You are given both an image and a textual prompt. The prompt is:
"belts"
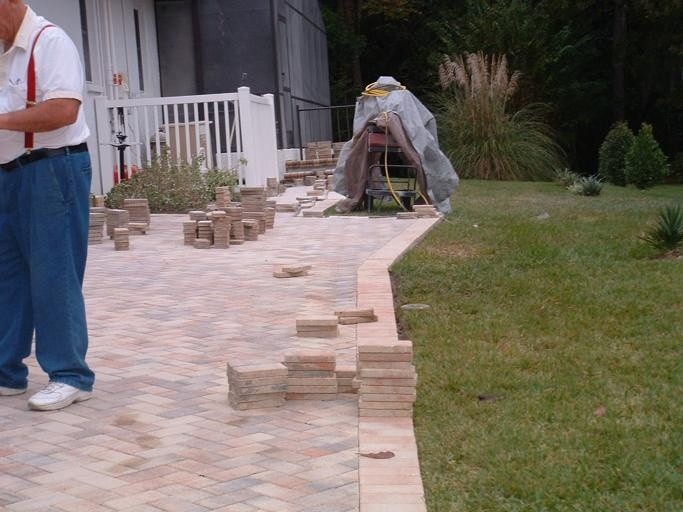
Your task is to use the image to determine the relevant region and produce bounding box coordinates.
[0,143,88,171]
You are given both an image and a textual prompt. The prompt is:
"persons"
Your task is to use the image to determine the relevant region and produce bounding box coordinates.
[0,0,96,411]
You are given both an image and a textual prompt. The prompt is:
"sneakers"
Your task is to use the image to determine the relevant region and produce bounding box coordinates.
[0,386,26,396]
[27,382,94,411]
